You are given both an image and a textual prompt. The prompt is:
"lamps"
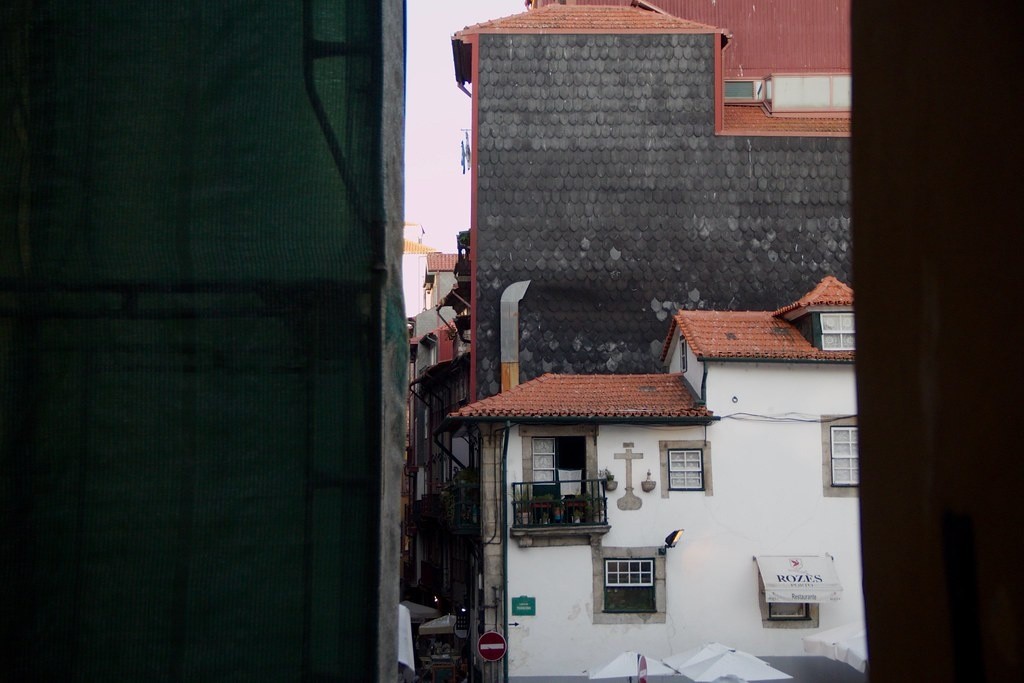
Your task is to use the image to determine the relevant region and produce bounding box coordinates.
[658,529,684,555]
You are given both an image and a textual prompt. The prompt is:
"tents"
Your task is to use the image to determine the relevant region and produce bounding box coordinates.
[418,615,457,635]
[399,600,440,623]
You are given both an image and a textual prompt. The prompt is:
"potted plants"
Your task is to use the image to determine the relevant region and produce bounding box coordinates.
[508,468,618,526]
[443,470,478,525]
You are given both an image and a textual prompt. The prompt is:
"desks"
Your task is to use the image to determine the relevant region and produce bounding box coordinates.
[431,661,455,683]
